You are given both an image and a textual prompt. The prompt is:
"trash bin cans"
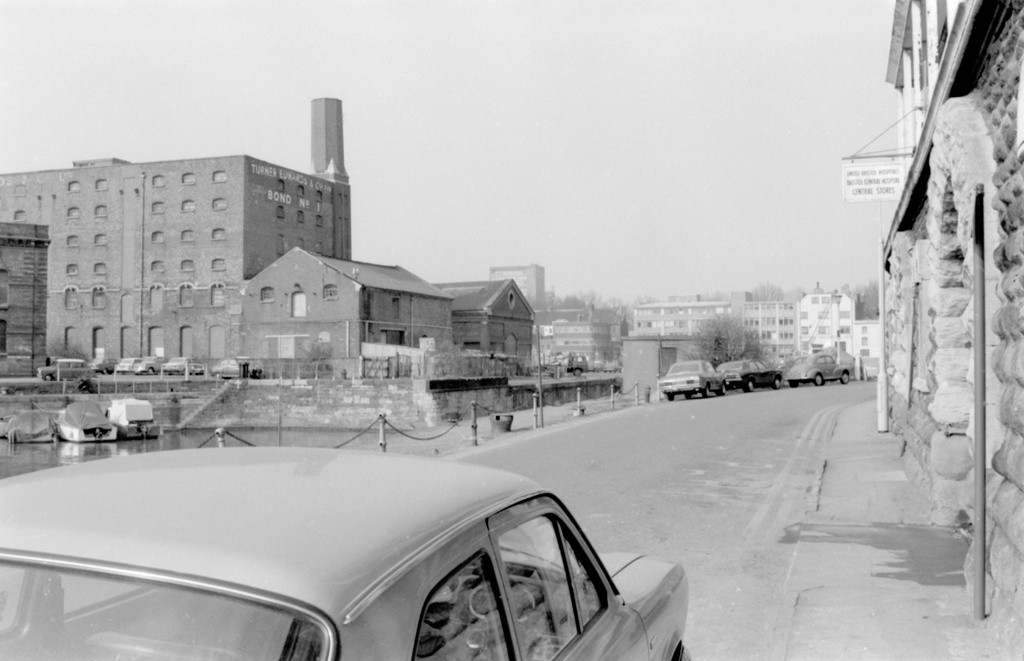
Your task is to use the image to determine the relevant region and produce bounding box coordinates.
[489,414,514,436]
[340,369,347,379]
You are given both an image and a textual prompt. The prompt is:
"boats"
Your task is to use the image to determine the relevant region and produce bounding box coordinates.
[4,409,57,449]
[53,400,119,442]
[104,398,160,440]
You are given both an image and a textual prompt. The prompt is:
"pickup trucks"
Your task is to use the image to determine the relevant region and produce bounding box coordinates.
[556,354,588,377]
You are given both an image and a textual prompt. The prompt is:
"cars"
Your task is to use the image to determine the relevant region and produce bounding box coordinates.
[36,358,94,381]
[1,445,689,660]
[91,357,118,374]
[602,362,617,374]
[784,354,852,388]
[210,357,259,381]
[658,360,726,401]
[716,359,784,393]
[114,357,145,376]
[162,357,206,374]
[141,356,171,374]
